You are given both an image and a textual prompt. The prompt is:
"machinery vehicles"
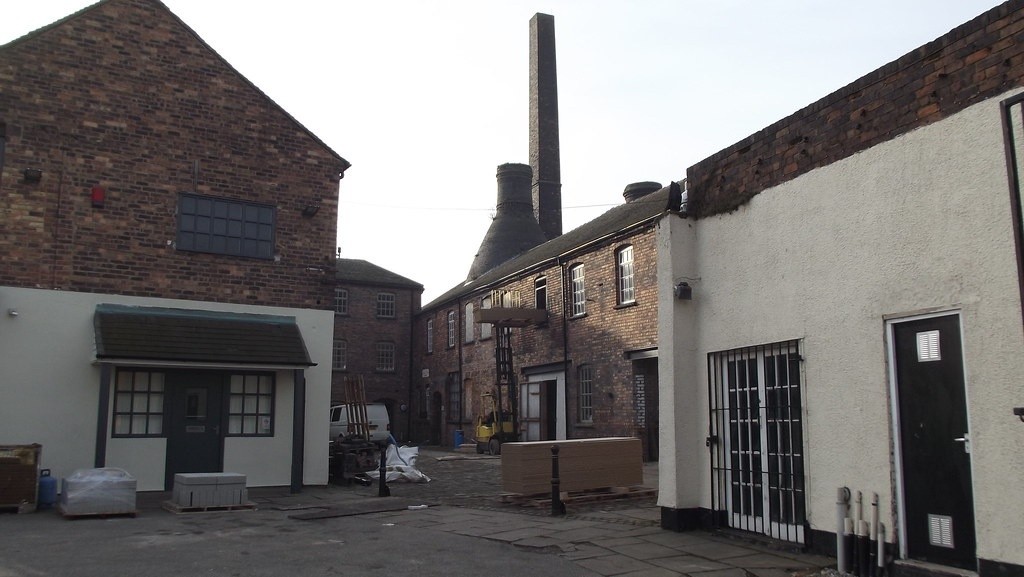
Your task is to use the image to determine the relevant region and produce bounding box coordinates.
[473,322,521,455]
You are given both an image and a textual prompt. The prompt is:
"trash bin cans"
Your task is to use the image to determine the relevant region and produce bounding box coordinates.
[454,429,464,449]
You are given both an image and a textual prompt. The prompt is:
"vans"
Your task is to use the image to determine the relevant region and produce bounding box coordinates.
[329,404,390,448]
